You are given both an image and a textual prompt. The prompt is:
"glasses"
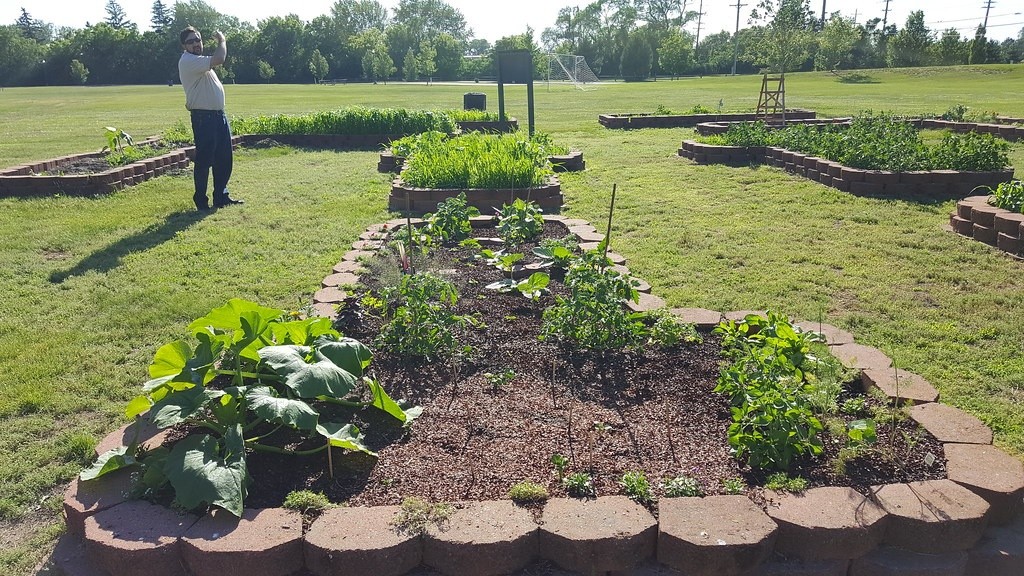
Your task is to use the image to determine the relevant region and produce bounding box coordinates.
[184,38,202,44]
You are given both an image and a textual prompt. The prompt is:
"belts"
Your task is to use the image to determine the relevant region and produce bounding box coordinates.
[191,110,224,115]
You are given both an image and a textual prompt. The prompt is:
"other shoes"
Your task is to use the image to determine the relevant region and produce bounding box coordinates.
[228,199,243,205]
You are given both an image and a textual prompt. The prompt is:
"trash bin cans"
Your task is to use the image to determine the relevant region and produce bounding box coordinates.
[475,78,479,83]
[464,92,486,111]
[168,81,173,86]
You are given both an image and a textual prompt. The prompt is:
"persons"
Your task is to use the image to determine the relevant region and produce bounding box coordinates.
[177,25,243,209]
